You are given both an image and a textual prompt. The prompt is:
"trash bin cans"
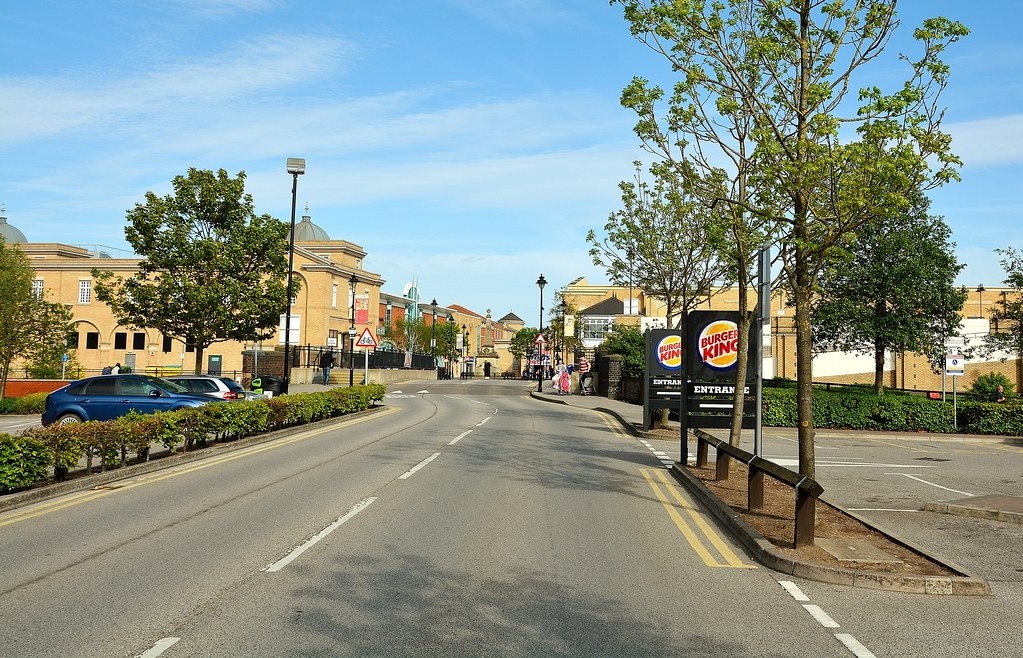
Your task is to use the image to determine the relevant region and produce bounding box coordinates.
[251,375,283,397]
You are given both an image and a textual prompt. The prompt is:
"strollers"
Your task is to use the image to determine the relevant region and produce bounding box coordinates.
[578,371,598,396]
[558,372,572,395]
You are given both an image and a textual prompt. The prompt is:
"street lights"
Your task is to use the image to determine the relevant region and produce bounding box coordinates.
[281,156,306,397]
[449,314,455,380]
[465,332,470,380]
[536,273,548,393]
[462,324,466,380]
[429,297,438,370]
[347,272,361,388]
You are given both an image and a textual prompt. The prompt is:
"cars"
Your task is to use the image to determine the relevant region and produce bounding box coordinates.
[249,375,283,396]
[122,375,237,403]
[40,373,229,430]
[186,374,268,401]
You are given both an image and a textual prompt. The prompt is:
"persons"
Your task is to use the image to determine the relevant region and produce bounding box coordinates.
[990,385,1007,404]
[510,371,515,380]
[552,358,571,389]
[503,370,510,380]
[111,362,121,374]
[521,370,528,380]
[319,350,334,385]
[536,369,540,380]
[571,353,591,392]
